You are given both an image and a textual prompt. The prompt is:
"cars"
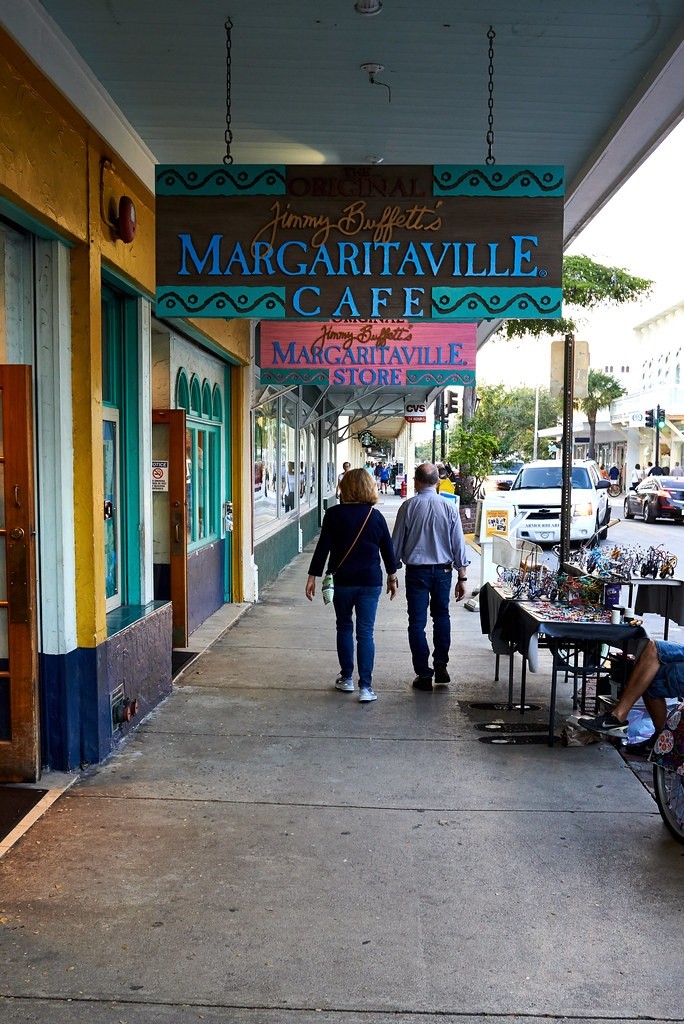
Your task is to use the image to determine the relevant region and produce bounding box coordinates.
[454,470,474,486]
[273,464,286,481]
[624,476,684,526]
[488,461,525,487]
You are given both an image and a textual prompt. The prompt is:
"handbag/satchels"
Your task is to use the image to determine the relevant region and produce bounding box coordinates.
[638,478,642,483]
[322,574,334,605]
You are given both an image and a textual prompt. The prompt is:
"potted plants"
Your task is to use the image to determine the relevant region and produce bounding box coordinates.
[446,429,499,533]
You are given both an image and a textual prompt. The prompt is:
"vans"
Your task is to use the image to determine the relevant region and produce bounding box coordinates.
[496,458,612,550]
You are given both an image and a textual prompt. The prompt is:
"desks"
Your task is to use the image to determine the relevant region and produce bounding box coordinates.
[468,561,681,747]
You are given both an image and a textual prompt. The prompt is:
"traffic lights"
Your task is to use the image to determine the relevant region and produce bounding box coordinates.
[645,409,653,428]
[658,409,665,428]
[444,414,449,423]
[447,390,458,414]
[434,416,440,430]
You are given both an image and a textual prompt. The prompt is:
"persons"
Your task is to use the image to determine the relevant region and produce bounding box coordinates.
[577,636,684,756]
[616,464,625,494]
[335,462,351,505]
[669,460,684,477]
[599,465,610,480]
[305,467,399,700]
[631,464,643,493]
[661,451,670,476]
[607,463,619,495]
[282,461,293,513]
[298,462,306,497]
[362,461,395,494]
[437,462,456,498]
[644,462,654,476]
[644,444,651,466]
[390,463,469,688]
[648,461,663,477]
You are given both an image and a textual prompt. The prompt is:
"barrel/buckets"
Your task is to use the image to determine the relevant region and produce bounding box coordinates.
[608,651,636,702]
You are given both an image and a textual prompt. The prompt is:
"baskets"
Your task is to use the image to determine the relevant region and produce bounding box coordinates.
[492,533,543,569]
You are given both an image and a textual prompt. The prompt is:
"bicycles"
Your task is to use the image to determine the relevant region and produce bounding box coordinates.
[604,477,621,497]
[551,518,621,675]
[646,702,684,842]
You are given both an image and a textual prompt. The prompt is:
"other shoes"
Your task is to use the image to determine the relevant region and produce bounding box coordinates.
[435,667,451,683]
[412,673,433,691]
[381,491,383,494]
[385,492,387,494]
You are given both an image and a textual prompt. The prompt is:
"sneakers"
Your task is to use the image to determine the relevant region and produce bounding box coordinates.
[358,686,378,701]
[578,712,630,737]
[625,732,662,756]
[335,676,355,691]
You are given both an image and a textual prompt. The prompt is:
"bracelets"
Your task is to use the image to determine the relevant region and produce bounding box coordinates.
[457,575,468,581]
[387,577,397,582]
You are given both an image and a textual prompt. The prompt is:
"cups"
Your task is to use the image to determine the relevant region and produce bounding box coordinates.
[611,610,620,624]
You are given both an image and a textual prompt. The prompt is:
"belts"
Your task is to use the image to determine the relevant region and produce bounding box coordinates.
[410,564,452,570]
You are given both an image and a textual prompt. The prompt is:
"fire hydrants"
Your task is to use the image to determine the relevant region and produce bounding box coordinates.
[400,481,407,498]
